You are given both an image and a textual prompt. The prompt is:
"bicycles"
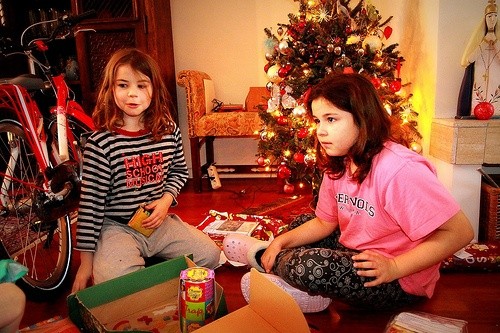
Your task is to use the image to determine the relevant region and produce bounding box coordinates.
[0,9,99,302]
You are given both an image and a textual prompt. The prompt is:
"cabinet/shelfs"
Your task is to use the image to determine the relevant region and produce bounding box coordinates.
[429,117,500,165]
[26,0,179,132]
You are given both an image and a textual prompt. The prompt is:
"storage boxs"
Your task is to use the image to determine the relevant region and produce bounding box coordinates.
[481,174,500,241]
[66,254,311,333]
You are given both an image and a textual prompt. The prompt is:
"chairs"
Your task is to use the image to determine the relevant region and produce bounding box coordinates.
[177,70,273,193]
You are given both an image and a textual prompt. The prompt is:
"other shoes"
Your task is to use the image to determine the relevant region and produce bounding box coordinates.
[223,233,270,273]
[240,272,330,314]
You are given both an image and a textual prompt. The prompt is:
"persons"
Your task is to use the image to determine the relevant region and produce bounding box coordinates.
[223,72,474,312]
[71,47,221,295]
[455,0,500,120]
[0,283,26,333]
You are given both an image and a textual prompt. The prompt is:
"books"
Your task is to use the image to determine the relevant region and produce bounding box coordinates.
[203,220,260,237]
